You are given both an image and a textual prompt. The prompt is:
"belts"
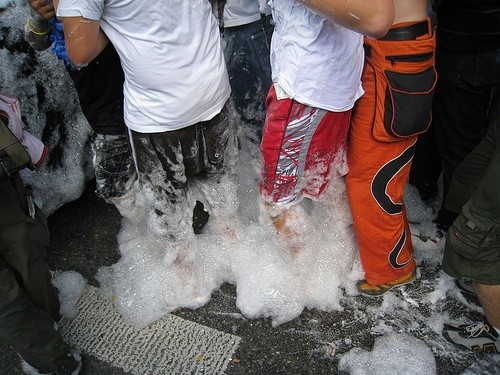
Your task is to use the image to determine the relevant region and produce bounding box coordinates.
[377,18,436,40]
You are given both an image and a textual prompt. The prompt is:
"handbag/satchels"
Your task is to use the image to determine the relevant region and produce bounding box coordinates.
[370,48,437,142]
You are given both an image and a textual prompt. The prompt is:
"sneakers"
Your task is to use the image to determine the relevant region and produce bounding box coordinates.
[441,315,500,353]
[455,278,482,308]
[356,266,417,296]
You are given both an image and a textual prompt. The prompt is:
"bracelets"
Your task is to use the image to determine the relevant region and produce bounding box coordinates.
[27,18,51,36]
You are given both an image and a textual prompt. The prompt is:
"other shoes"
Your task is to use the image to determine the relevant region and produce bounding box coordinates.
[52,271,84,324]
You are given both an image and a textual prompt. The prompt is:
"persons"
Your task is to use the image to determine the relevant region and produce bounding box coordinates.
[207,1,276,146]
[258,1,394,254]
[0,120,82,375]
[25,0,138,203]
[56,1,241,242]
[410,0,500,233]
[442,101,500,354]
[344,1,437,296]
